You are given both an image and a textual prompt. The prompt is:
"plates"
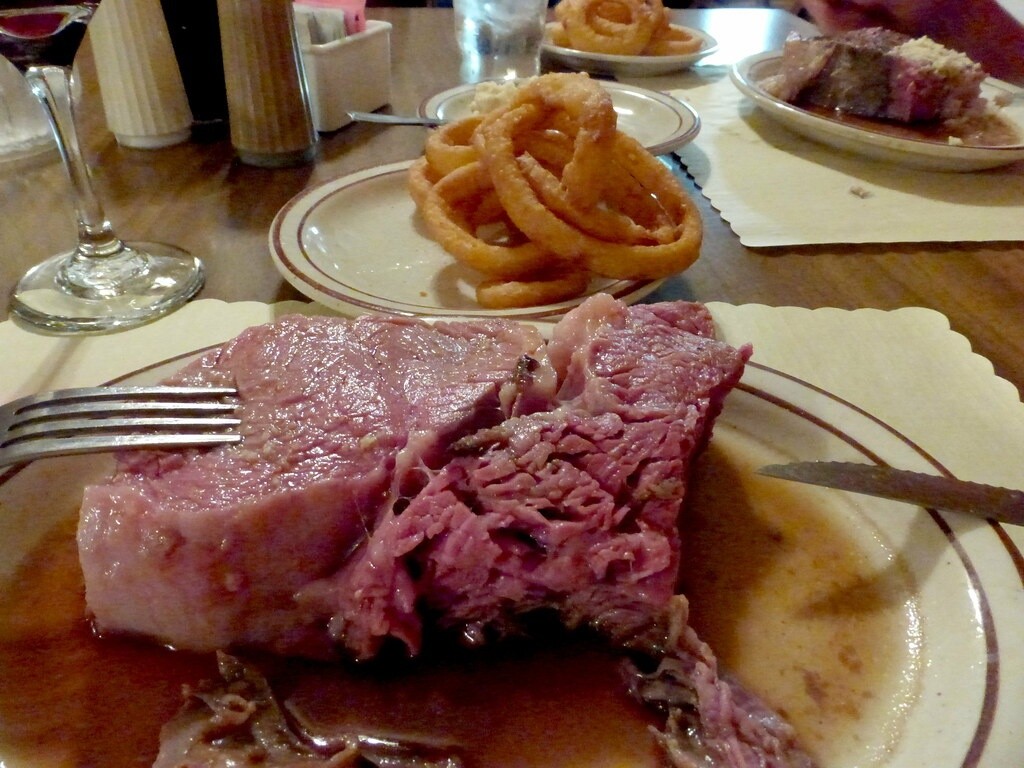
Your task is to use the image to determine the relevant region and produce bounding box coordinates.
[728,48,1024,174]
[418,81,701,161]
[0,328,1024,768]
[541,27,721,74]
[269,158,679,330]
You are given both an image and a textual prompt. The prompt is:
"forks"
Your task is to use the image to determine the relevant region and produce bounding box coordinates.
[1,379,245,485]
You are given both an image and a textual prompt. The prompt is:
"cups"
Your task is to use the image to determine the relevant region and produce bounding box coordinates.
[451,0,548,85]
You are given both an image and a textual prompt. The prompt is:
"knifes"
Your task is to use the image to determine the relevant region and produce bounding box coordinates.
[752,459,1024,531]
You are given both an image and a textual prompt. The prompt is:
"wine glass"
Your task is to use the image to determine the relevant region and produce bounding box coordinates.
[0,0,205,331]
